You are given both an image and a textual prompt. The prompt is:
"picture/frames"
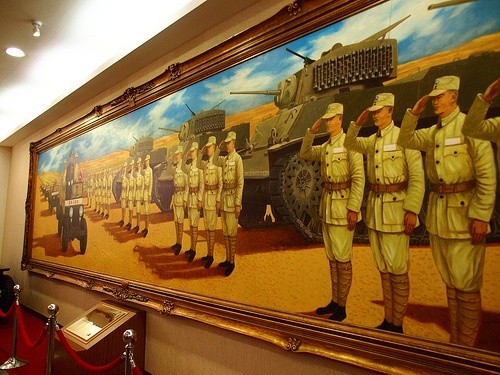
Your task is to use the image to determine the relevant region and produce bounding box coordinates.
[21,0,500,375]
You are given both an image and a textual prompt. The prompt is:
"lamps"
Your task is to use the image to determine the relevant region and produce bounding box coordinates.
[32,21,42,37]
